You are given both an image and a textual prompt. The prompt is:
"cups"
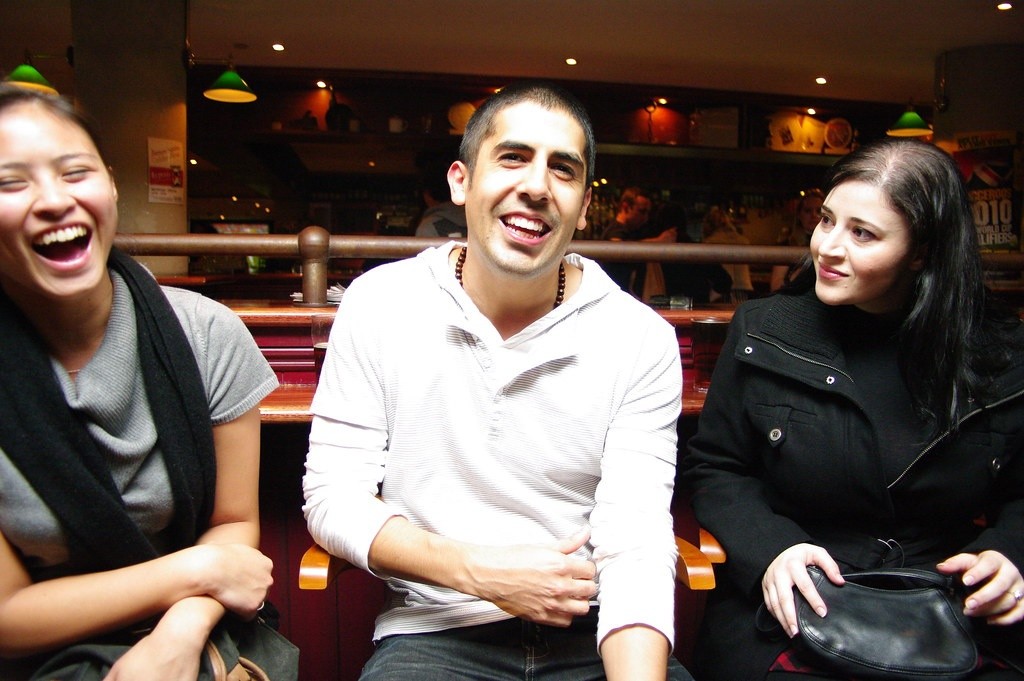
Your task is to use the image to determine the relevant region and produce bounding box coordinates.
[689,317,732,394]
[311,315,335,385]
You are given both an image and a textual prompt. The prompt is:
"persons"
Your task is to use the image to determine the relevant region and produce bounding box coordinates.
[700,211,754,303]
[770,189,825,292]
[304,86,696,681]
[686,139,1024,681]
[415,165,467,238]
[0,87,278,681]
[189,221,248,273]
[596,188,677,293]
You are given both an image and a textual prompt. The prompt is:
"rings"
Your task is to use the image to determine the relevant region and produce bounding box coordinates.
[257,602,264,610]
[1006,591,1023,607]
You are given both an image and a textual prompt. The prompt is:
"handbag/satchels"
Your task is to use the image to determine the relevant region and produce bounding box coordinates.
[31,615,300,681]
[756,559,979,681]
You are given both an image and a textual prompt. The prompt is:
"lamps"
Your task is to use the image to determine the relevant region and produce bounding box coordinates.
[190,55,258,103]
[886,96,936,137]
[2,44,74,98]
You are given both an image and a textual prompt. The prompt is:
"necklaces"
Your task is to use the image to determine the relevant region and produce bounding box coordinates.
[68,370,80,373]
[455,246,566,307]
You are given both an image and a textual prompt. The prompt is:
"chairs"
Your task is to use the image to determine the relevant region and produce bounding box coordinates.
[296,518,731,680]
